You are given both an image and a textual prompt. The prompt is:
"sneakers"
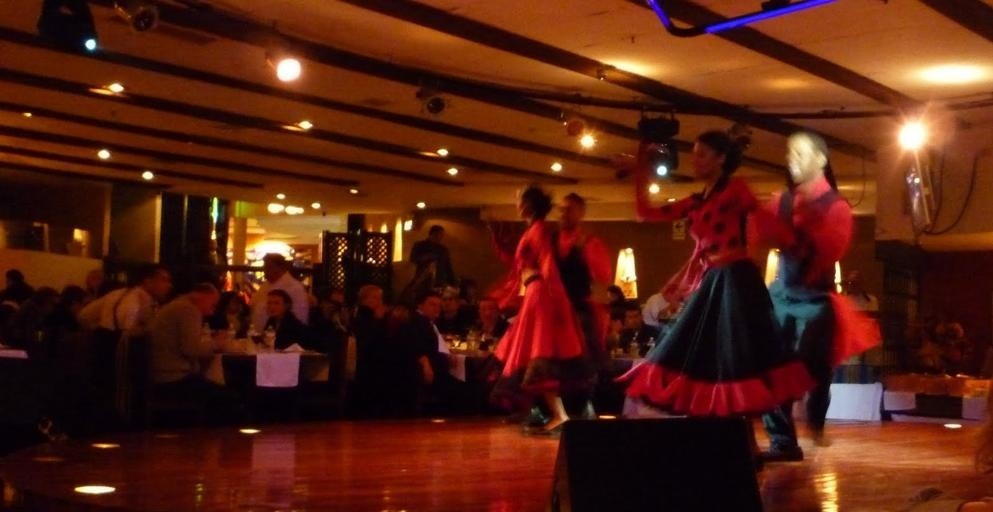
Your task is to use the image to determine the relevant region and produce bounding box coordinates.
[758,443,803,461]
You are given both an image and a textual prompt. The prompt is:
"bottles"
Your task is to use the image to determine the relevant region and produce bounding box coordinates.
[442,327,493,351]
[627,331,656,357]
[217,322,276,350]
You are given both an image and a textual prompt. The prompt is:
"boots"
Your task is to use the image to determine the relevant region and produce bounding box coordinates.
[542,396,571,430]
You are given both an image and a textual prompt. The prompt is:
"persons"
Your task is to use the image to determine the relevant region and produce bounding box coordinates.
[1,224,513,464]
[493,128,881,462]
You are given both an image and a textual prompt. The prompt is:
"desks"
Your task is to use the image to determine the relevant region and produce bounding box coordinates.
[214,338,326,424]
[445,348,492,383]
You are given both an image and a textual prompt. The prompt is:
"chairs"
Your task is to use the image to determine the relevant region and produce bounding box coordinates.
[115,328,205,432]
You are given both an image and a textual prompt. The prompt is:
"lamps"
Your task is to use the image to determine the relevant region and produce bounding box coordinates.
[113,0,160,34]
[262,35,301,83]
[636,111,679,177]
[416,87,447,116]
[560,109,584,136]
